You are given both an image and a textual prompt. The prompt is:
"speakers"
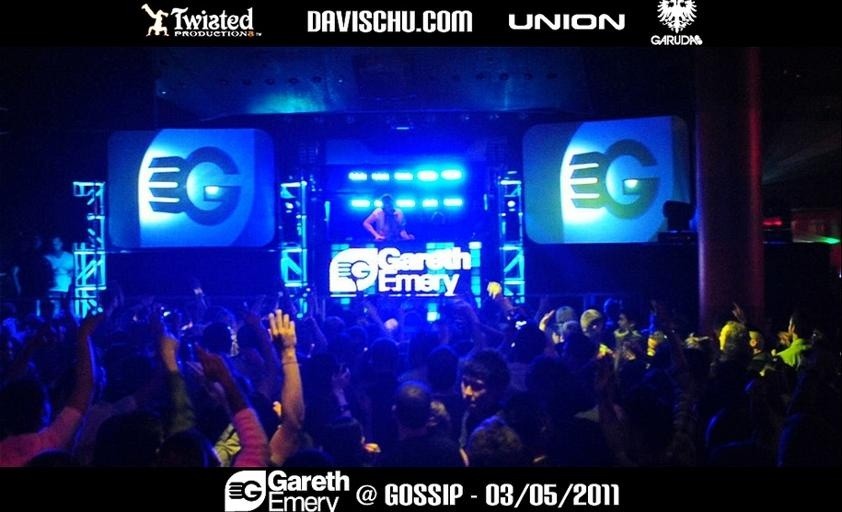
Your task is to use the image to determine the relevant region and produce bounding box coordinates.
[657,229,699,336]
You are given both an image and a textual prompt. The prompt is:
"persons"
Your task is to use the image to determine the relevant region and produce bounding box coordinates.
[45,236,77,301]
[363,194,416,241]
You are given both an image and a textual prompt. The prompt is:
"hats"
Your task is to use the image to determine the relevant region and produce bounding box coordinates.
[580,309,602,328]
